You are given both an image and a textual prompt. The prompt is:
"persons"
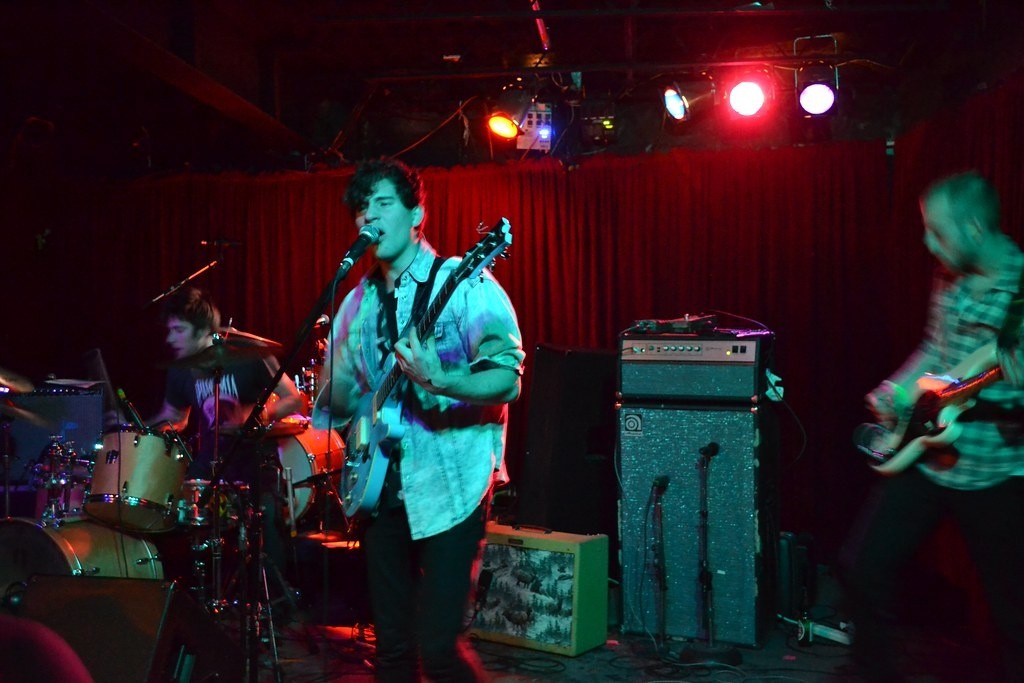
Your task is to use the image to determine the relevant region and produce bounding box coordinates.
[833,170,1024,683]
[158,286,303,644]
[312,158,526,683]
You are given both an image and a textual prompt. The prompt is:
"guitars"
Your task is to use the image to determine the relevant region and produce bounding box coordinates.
[340,216,515,523]
[865,364,1005,475]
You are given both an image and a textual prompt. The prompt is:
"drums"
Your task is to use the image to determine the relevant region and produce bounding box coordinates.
[0,514,166,603]
[84,424,192,536]
[256,415,348,535]
[181,479,245,527]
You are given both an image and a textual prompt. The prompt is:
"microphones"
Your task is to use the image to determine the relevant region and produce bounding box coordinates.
[201,241,242,250]
[336,224,379,280]
[284,468,297,538]
[653,476,669,488]
[117,388,148,433]
[699,442,720,456]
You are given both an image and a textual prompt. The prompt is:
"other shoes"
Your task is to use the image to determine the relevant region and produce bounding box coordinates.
[774,592,863,647]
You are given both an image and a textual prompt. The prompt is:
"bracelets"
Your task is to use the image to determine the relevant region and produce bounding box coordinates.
[883,380,906,398]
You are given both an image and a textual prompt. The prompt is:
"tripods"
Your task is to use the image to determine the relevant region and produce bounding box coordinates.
[216,445,320,683]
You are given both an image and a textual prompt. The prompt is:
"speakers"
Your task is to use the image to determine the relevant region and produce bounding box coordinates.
[466,518,609,656]
[0,383,105,486]
[614,401,781,650]
[517,343,621,587]
[17,573,244,683]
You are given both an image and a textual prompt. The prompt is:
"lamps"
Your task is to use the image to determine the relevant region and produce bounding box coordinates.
[485,77,540,139]
[796,68,836,116]
[730,70,776,116]
[663,72,721,121]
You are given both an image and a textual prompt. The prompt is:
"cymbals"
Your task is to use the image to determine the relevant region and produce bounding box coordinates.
[216,325,284,351]
